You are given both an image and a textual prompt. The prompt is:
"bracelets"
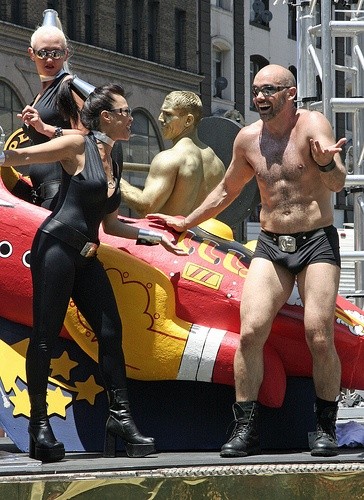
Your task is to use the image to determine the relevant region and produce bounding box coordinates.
[52,126,64,140]
[0,152,6,166]
[320,159,337,173]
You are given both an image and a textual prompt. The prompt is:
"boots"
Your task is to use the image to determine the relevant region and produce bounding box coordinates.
[27,392,66,460]
[310,398,339,457]
[101,386,158,458]
[219,402,263,457]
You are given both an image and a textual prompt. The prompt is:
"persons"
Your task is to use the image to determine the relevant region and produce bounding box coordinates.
[115,90,224,219]
[145,65,347,456]
[0,24,192,462]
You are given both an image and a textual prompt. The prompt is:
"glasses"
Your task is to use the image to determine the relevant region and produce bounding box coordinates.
[110,106,132,118]
[253,85,294,97]
[29,44,70,60]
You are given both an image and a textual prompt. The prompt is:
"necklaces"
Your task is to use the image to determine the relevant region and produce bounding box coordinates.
[103,144,114,190]
